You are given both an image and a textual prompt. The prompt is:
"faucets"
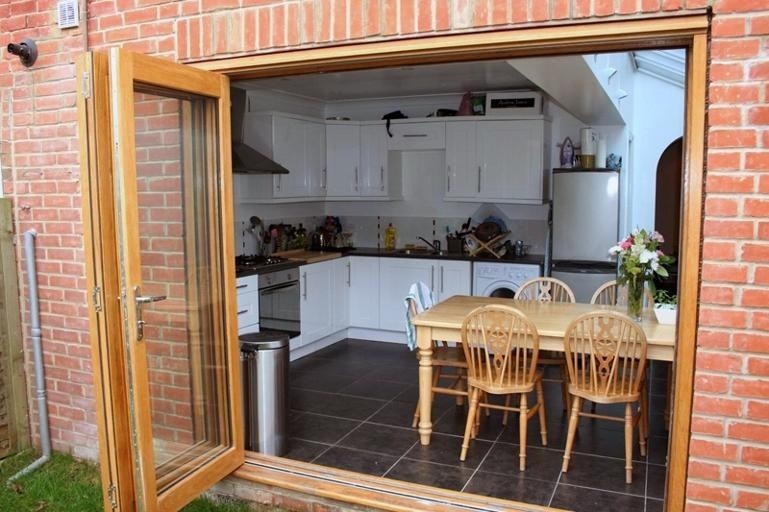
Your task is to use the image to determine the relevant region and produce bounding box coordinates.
[417,236,440,253]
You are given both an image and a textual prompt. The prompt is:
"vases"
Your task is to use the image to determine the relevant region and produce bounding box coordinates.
[625,278,643,323]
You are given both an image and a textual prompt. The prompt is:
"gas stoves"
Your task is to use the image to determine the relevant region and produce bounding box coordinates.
[235,254,299,288]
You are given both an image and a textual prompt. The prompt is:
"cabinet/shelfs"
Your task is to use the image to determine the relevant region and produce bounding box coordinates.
[378,253,472,334]
[327,118,360,201]
[346,255,379,331]
[236,274,260,336]
[443,115,478,203]
[243,112,325,205]
[480,112,551,206]
[388,118,443,153]
[298,256,347,352]
[363,118,401,201]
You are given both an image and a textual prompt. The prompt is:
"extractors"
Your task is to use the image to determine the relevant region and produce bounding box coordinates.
[228,85,289,176]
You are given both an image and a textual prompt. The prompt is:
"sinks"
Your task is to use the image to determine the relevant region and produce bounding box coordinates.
[392,249,444,256]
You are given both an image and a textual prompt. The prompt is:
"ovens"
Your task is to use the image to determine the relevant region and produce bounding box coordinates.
[258,280,300,342]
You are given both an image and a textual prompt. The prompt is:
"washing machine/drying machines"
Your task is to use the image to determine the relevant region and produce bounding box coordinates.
[472,261,542,349]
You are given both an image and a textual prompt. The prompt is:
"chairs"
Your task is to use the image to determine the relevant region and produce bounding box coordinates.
[559,309,650,486]
[565,279,655,441]
[400,281,492,431]
[499,278,577,428]
[455,302,549,472]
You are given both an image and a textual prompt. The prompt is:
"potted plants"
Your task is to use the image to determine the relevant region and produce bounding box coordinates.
[651,288,678,328]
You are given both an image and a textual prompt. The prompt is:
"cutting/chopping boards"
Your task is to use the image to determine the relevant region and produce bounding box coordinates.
[271,249,342,263]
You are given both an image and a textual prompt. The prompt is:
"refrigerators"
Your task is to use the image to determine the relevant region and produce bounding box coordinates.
[549,167,620,306]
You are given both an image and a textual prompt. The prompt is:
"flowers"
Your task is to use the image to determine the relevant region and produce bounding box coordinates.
[607,224,677,316]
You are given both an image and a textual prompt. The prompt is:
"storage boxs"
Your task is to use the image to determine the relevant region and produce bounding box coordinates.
[485,91,549,116]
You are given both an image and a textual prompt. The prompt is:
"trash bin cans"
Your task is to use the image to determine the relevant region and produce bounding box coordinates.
[239,332,289,457]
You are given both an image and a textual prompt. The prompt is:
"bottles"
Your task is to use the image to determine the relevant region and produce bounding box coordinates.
[458,91,472,116]
[280,231,288,250]
[515,240,523,256]
